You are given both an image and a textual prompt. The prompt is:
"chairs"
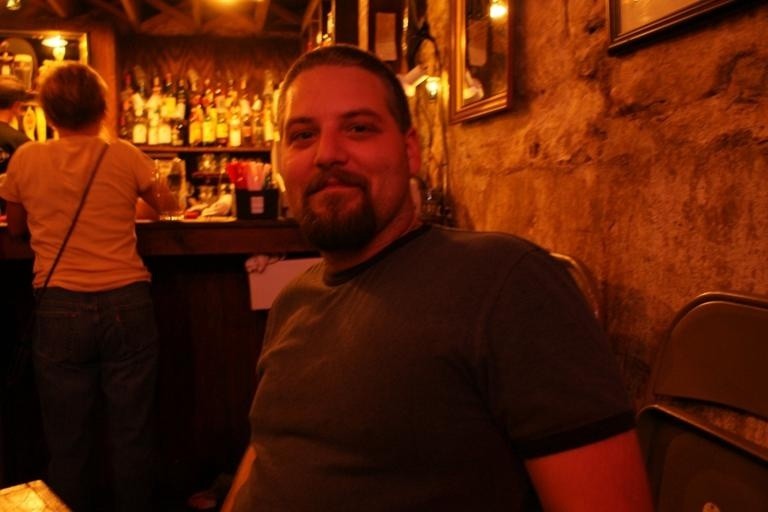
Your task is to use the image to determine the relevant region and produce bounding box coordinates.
[637,290,768,511]
[554,251,604,329]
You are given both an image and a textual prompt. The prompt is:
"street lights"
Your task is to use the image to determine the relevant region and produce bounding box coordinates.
[2,301,37,388]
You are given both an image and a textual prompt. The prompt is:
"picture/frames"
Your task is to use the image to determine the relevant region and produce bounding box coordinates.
[0,27,90,107]
[447,0,513,126]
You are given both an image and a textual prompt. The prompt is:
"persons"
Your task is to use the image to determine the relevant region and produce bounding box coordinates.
[2,66,180,512]
[221,42,654,512]
[2,74,32,173]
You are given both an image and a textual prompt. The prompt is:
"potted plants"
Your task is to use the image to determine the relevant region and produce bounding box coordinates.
[0,27,90,107]
[447,0,513,126]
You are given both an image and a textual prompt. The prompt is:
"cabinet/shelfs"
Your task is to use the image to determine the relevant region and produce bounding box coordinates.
[122,69,271,215]
[13,55,34,91]
